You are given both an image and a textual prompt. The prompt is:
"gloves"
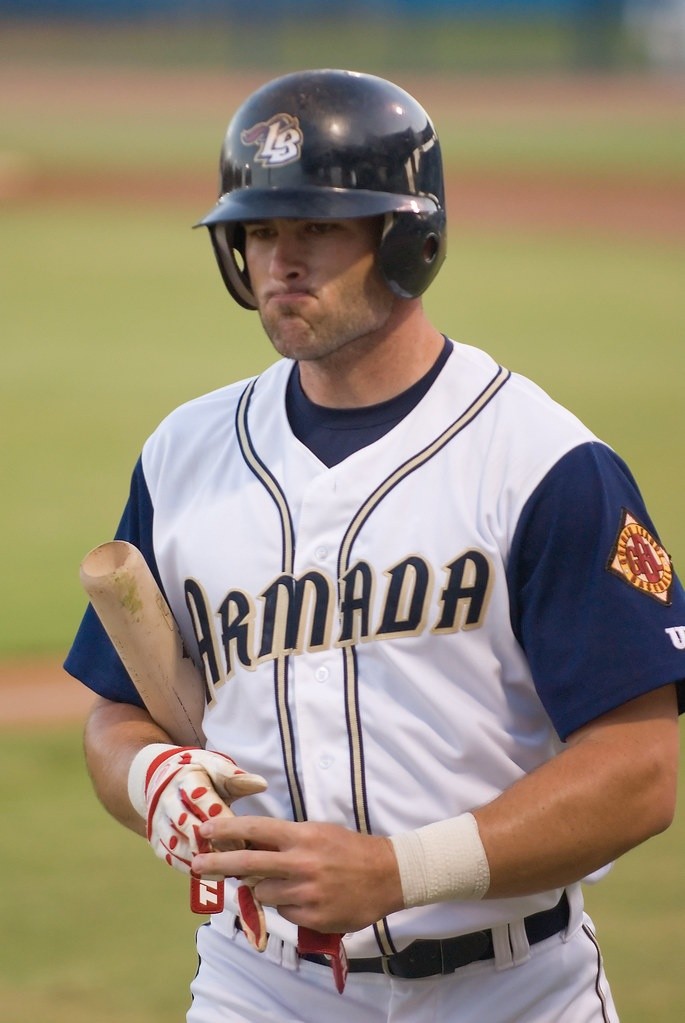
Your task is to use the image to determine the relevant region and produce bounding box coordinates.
[128,743,267,882]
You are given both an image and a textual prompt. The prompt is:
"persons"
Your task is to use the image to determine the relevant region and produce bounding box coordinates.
[64,69,685,1023]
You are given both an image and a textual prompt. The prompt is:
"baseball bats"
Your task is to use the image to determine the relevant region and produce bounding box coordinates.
[76,538,211,751]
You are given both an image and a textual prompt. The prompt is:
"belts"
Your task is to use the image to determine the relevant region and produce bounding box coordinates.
[235,889,571,977]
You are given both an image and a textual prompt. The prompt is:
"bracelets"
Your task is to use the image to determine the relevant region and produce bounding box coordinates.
[386,812,491,909]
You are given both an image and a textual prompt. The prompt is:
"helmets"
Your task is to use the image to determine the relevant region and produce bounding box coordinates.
[191,66,447,312]
